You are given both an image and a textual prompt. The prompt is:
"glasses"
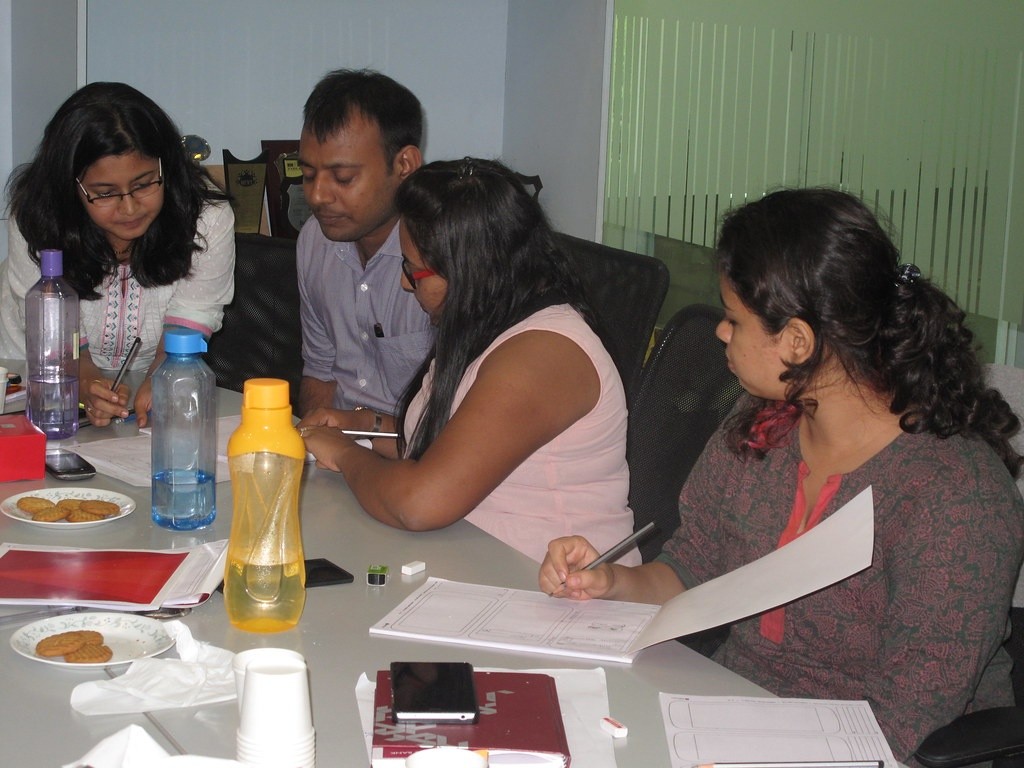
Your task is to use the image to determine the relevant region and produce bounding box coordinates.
[74,155,163,208]
[400,258,435,289]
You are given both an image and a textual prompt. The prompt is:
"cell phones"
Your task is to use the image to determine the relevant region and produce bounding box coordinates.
[217,558,354,594]
[390,662,479,726]
[43,447,96,480]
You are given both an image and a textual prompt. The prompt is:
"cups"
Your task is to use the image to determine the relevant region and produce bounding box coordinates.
[231,647,317,768]
[0,367,8,414]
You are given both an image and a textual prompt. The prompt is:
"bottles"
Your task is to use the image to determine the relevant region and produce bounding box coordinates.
[223,377,307,634]
[24,248,80,440]
[151,328,217,532]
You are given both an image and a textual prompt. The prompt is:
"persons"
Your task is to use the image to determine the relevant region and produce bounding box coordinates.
[0,82,234,424]
[540,189,1024,768]
[295,71,431,413]
[295,156,642,567]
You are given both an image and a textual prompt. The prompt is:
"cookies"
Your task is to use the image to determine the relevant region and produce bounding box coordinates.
[17,496,119,523]
[36,630,114,663]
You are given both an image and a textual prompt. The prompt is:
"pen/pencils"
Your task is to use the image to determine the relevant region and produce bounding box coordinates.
[111,337,141,392]
[342,430,398,437]
[374,323,384,338]
[115,411,151,424]
[692,760,884,768]
[549,522,655,595]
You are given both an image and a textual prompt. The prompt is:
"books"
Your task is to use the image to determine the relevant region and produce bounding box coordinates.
[371,669,571,768]
[368,484,874,664]
[0,540,227,611]
[138,414,373,472]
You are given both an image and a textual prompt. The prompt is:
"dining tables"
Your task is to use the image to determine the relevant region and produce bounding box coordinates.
[0,360,912,768]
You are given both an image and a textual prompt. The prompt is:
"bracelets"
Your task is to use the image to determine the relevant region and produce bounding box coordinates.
[368,411,382,439]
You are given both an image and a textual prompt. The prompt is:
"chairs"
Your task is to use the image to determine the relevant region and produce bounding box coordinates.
[552,230,669,384]
[913,607,1024,768]
[620,303,747,658]
[200,232,304,417]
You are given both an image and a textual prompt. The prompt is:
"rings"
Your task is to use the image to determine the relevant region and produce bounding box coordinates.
[300,428,304,436]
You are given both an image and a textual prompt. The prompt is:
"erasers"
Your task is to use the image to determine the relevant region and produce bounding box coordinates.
[600,717,628,738]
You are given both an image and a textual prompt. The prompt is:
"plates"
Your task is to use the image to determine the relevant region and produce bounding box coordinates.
[10,612,176,672]
[0,487,137,530]
